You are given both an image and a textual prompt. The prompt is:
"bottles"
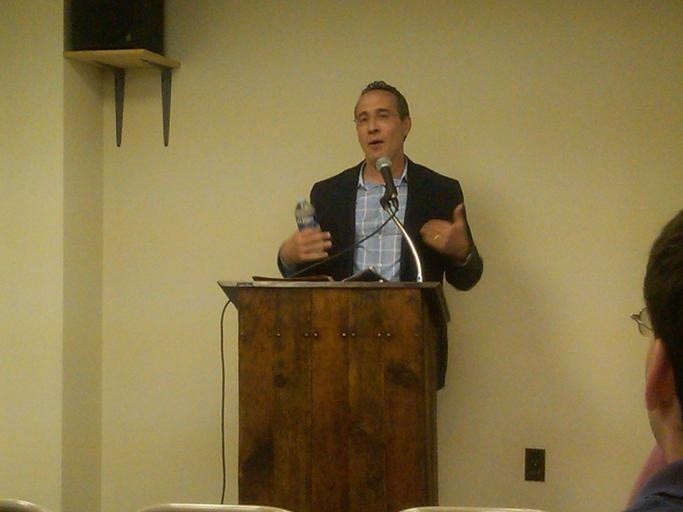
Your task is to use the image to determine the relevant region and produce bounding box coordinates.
[293,196,320,231]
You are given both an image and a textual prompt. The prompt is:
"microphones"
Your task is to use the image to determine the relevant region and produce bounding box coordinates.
[375,156,399,209]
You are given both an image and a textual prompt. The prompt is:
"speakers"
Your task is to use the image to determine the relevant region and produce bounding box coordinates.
[71,0,165,54]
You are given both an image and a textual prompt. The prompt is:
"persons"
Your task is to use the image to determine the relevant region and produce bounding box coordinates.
[618,208,683,510]
[272,80,483,294]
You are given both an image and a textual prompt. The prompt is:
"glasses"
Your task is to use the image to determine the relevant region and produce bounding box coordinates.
[628,305,655,339]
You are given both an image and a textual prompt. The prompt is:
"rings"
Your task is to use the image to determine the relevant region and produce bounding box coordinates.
[433,234,441,240]
